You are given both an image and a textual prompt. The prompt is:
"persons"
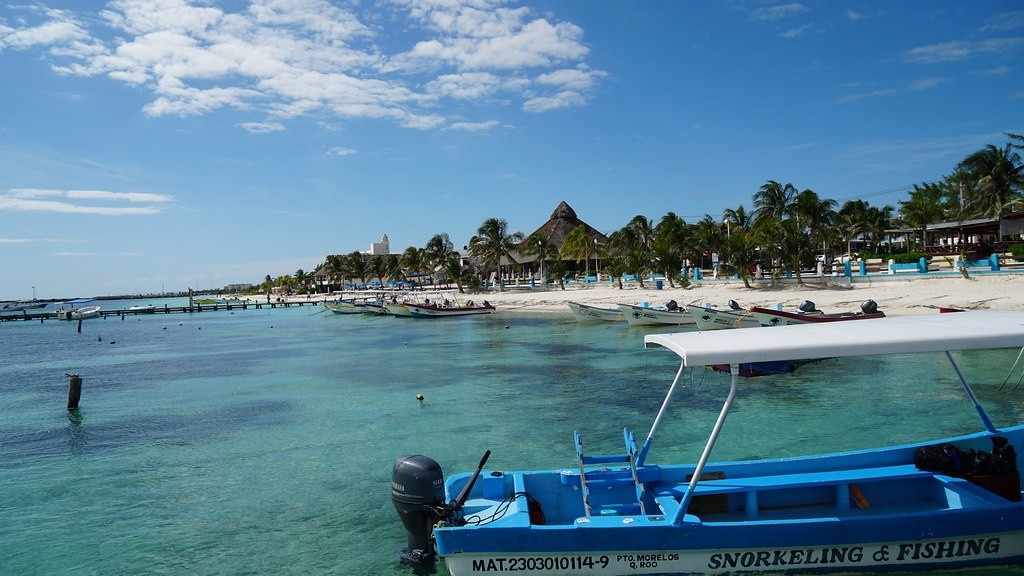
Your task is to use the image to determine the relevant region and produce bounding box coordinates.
[412,282,414,289]
[307,290,311,302]
[267,291,270,303]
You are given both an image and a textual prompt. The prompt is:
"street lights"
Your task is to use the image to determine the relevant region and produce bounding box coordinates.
[538,241,543,284]
[594,238,599,273]
[725,219,730,241]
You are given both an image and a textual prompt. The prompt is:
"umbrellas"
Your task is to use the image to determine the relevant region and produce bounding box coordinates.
[389,281,409,289]
[345,281,381,289]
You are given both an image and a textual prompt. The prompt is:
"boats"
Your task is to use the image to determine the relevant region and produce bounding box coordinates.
[398,310,1024,576]
[193,297,252,304]
[130,303,155,310]
[404,292,496,318]
[323,292,455,316]
[565,299,687,323]
[685,299,823,331]
[616,298,746,326]
[0,302,48,312]
[54,299,94,305]
[56,306,101,320]
[747,299,886,325]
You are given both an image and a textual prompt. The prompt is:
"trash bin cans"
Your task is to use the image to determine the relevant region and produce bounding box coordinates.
[657,281,662,289]
